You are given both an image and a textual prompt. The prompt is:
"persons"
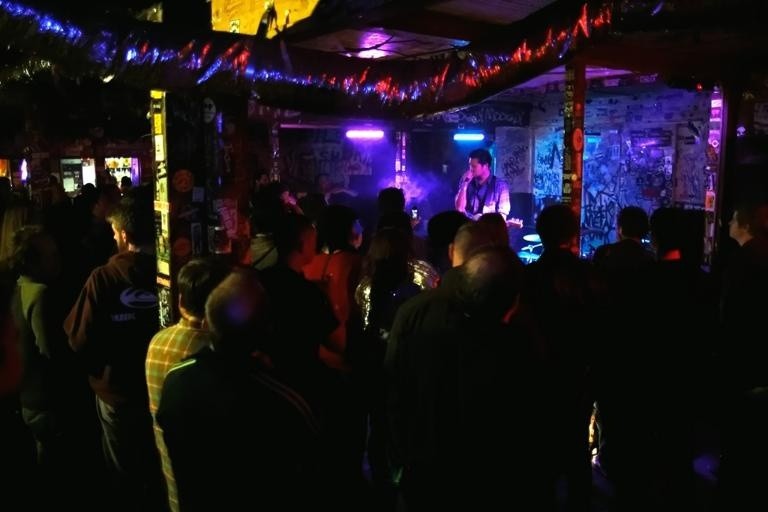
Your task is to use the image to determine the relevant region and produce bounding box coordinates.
[1,167,768,510]
[454,148,512,224]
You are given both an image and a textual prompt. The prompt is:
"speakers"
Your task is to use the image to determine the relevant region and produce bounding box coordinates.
[510,192,535,225]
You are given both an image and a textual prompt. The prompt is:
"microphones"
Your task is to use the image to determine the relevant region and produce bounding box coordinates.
[465,177,469,182]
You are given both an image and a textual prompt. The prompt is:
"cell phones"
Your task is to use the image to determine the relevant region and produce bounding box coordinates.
[410,207,420,221]
